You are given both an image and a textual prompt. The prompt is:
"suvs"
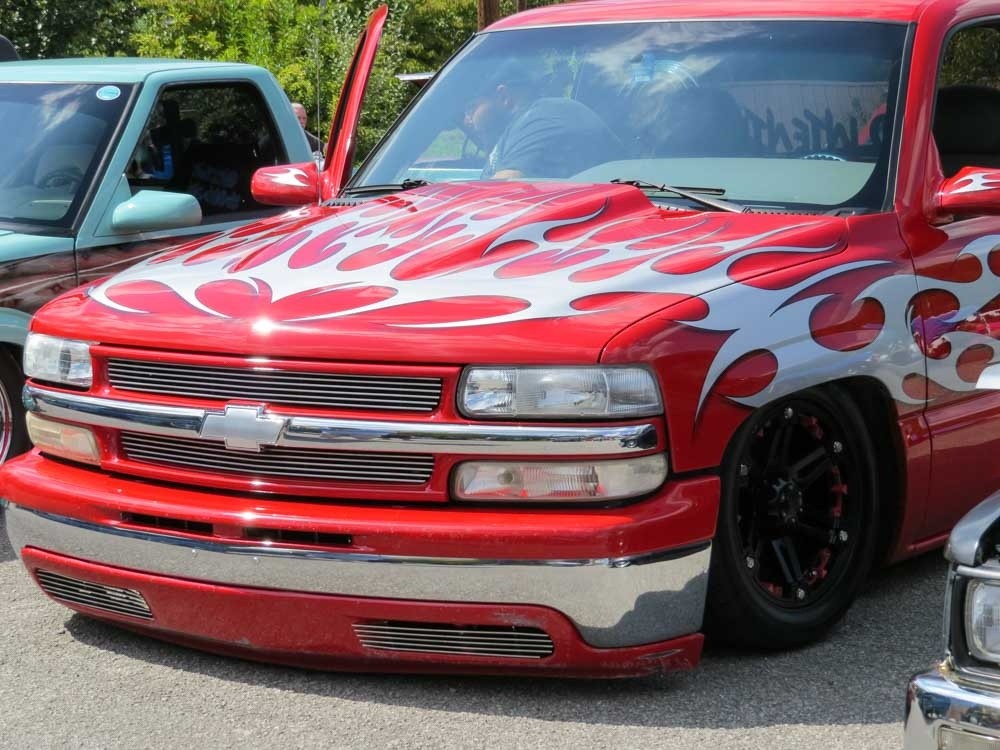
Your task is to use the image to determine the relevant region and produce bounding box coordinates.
[1,0,999,682]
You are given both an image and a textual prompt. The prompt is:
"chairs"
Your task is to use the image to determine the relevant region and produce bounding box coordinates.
[931,83,1000,176]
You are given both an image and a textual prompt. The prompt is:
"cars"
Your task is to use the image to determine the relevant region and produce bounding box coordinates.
[903,489,999,750]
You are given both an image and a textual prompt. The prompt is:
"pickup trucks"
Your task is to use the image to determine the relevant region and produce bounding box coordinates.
[0,59,361,462]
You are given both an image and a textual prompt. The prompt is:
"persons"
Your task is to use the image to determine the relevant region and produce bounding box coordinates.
[290,102,328,157]
[457,61,629,179]
